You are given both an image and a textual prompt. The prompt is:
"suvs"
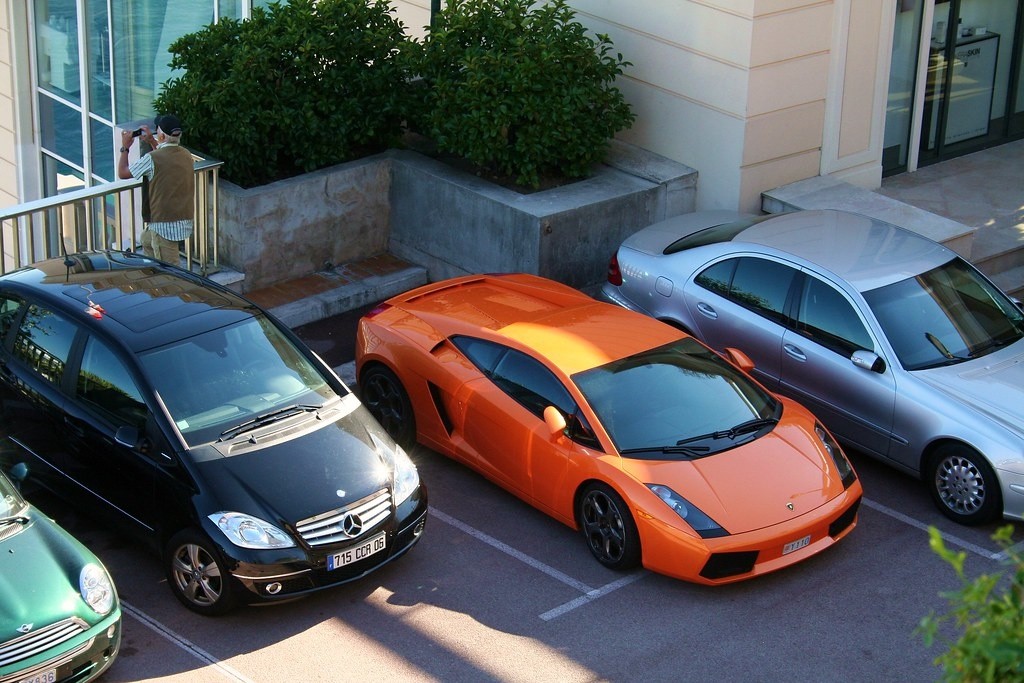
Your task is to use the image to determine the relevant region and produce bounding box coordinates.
[0,249,430,617]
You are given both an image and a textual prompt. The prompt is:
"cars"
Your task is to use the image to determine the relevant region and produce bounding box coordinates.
[0,458,124,683]
[601,209,1024,526]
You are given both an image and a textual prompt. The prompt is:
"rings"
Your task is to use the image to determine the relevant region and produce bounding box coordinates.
[123,132,127,135]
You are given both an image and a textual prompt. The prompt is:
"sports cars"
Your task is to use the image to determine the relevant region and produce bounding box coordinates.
[355,273,864,586]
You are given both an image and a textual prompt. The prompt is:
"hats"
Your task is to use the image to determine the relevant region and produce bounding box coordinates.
[154,115,181,136]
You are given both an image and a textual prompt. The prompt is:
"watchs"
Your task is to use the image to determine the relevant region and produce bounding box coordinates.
[120,146,129,153]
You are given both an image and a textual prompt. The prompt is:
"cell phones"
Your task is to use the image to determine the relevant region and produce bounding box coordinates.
[132,128,142,137]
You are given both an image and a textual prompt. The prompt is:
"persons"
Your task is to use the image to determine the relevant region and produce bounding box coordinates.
[118,114,194,267]
